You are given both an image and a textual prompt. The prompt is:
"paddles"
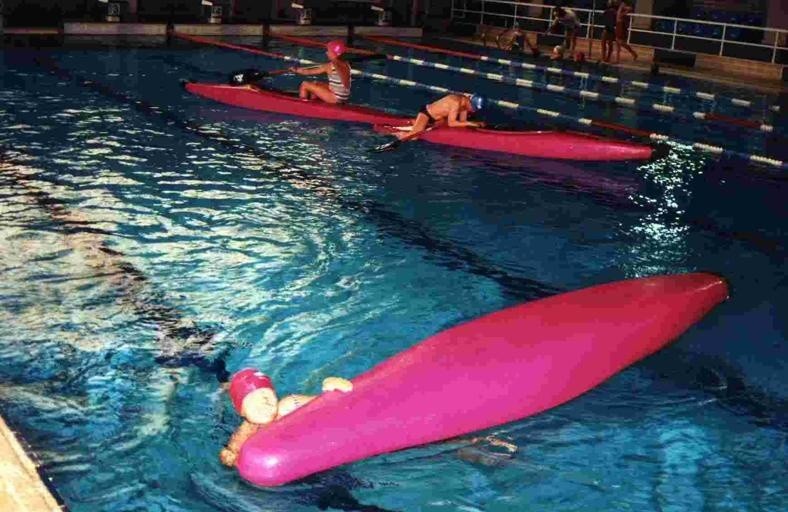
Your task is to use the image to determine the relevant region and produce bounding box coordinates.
[372,117,443,152]
[228,61,326,87]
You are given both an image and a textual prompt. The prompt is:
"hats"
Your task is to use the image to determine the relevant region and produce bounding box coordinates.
[229,368,275,416]
[469,92,486,112]
[328,40,346,57]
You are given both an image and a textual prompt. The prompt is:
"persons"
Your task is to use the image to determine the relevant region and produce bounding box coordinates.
[494,0,639,64]
[288,40,352,104]
[370,93,489,153]
[220,368,353,468]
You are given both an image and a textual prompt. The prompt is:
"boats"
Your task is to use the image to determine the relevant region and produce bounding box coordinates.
[179,79,485,137]
[237,270,730,488]
[372,121,656,169]
